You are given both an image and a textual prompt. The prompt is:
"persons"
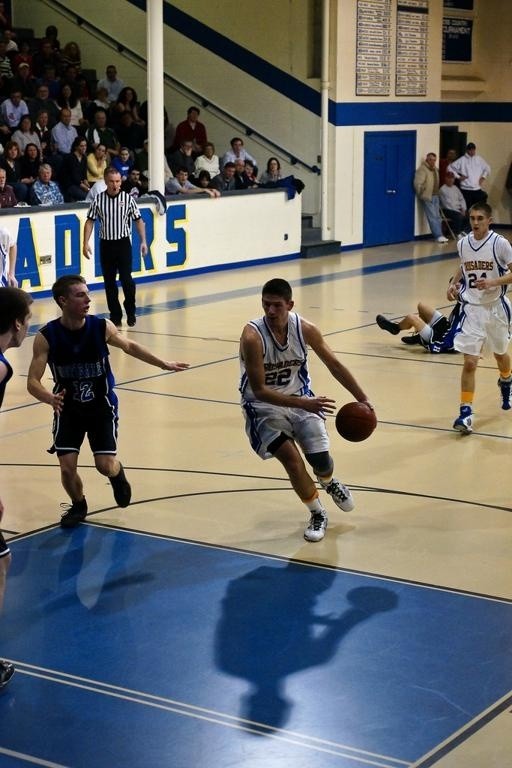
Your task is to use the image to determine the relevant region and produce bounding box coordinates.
[1,226,17,291]
[83,167,148,328]
[1,289,33,688]
[28,276,190,527]
[414,142,490,242]
[377,276,465,352]
[447,205,511,434]
[240,279,377,542]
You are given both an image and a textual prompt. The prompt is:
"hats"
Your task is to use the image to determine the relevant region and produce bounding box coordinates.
[18,62,29,68]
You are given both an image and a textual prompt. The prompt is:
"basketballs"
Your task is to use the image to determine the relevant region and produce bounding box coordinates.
[336,401,377,443]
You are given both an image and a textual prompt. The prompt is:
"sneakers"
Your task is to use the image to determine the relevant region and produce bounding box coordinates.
[106,462,131,508]
[452,405,474,433]
[127,313,135,327]
[317,477,355,512]
[304,508,329,541]
[109,318,120,325]
[401,334,420,345]
[435,236,449,242]
[498,376,512,409]
[59,499,88,527]
[377,315,400,335]
[0,659,15,687]
